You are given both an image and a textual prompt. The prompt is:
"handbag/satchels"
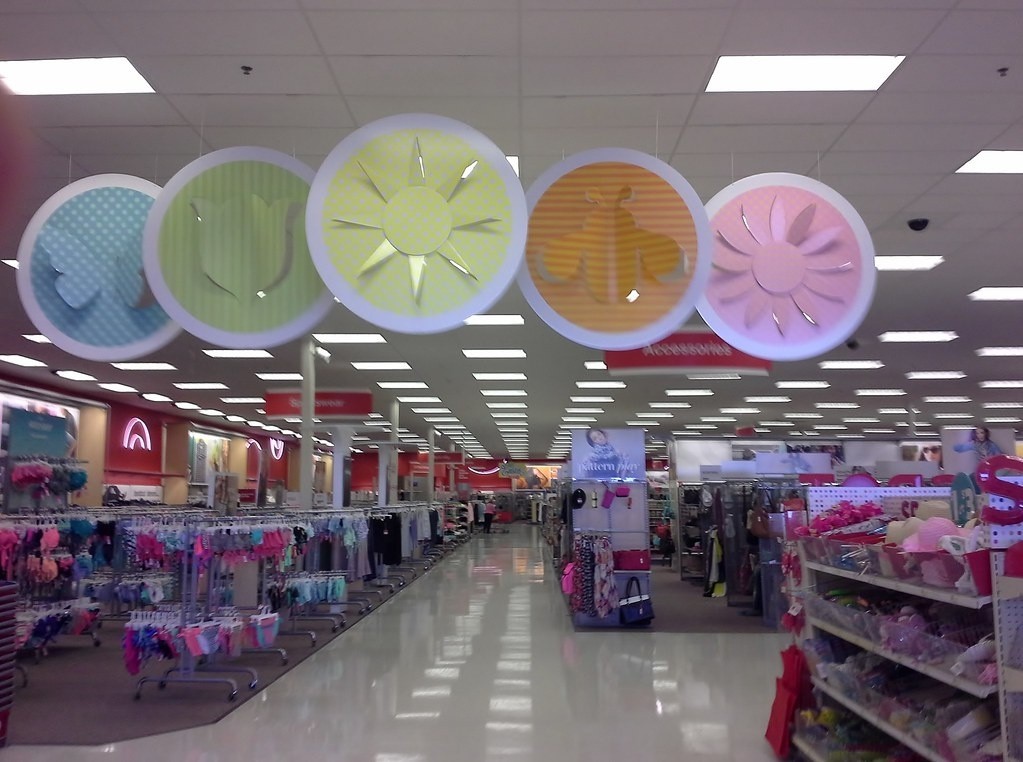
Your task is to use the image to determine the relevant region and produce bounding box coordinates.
[750,490,783,540]
[620,576,654,625]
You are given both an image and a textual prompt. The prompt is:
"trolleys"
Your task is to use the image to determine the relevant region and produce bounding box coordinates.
[489,513,510,534]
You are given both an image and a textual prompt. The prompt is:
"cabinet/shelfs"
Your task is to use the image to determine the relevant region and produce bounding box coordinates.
[792,487,1023,762]
[546,482,791,632]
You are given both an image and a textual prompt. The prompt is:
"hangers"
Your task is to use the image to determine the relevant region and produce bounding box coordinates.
[1,504,436,632]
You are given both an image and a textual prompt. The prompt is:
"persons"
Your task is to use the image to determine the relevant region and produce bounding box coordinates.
[918,444,943,470]
[520,467,548,489]
[585,429,626,477]
[954,428,1002,462]
[483,500,495,534]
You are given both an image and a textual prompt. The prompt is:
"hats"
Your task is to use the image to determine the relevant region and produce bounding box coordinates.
[886,497,952,546]
[903,516,971,553]
